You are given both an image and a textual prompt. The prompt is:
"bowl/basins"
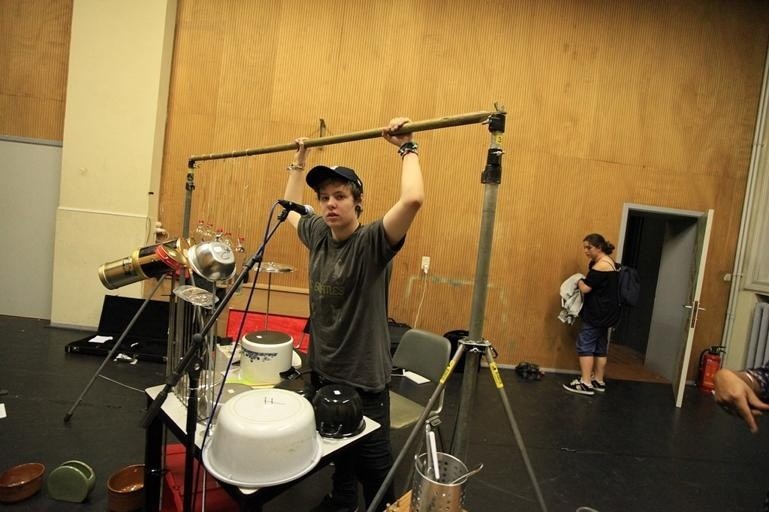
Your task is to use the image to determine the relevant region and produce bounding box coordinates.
[175,370,225,401]
[0,462,47,501]
[197,382,253,427]
[106,463,150,500]
[200,386,324,489]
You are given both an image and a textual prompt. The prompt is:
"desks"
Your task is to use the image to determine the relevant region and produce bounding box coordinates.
[142,368,382,512]
[385,486,468,512]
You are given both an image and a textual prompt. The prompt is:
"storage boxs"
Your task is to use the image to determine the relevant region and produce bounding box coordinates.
[162,443,241,511]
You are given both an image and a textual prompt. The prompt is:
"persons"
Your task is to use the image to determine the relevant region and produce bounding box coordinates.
[283,116,425,510]
[562,234,619,396]
[712,362,769,437]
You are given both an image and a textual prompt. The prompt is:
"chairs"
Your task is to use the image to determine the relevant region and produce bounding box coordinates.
[389,328,453,497]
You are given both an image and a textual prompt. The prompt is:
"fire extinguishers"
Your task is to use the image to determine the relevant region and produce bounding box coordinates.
[696,345,726,396]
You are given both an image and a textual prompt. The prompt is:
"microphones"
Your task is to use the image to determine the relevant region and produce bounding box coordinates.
[278,199,315,218]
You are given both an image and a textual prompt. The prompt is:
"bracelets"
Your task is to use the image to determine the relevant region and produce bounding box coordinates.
[286,161,305,172]
[397,141,420,157]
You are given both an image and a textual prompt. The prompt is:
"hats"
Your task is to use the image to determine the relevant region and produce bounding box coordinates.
[306,165,363,193]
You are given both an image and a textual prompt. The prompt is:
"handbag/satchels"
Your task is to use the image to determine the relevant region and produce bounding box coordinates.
[618,266,641,308]
[444,330,482,373]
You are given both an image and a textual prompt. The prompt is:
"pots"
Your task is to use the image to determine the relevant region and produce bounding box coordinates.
[188,239,237,281]
[312,383,366,439]
[272,379,315,404]
[238,328,302,385]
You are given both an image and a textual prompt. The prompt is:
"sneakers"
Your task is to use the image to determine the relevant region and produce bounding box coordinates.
[562,377,606,395]
[310,494,360,512]
